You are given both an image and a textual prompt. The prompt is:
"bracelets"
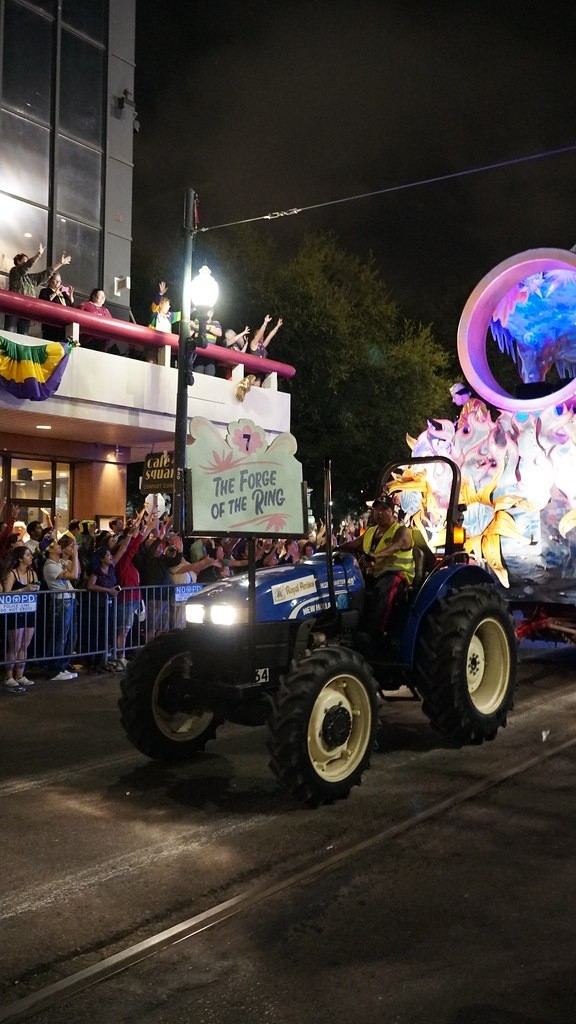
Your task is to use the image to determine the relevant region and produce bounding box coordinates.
[36,253,41,257]
[263,322,267,326]
[55,291,58,295]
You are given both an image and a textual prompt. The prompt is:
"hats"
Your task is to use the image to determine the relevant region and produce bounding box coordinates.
[449,382,466,396]
[372,496,394,508]
[163,532,179,544]
[39,538,57,557]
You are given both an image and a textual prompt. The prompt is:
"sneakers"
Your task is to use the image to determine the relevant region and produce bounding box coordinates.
[4,677,19,686]
[16,676,35,686]
[50,669,79,681]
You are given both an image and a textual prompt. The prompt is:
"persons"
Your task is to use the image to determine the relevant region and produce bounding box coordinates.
[0,496,372,686]
[147,282,182,363]
[38,272,73,342]
[190,306,223,377]
[78,289,113,354]
[449,383,487,432]
[250,314,283,388]
[330,496,415,640]
[218,326,250,382]
[2,243,71,335]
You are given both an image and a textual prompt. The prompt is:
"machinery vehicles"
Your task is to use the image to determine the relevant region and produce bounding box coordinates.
[111,456,576,806]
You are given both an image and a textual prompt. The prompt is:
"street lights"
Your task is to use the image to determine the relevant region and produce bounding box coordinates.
[165,190,221,563]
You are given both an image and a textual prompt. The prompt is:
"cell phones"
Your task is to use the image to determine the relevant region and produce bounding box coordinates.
[114,586,121,592]
